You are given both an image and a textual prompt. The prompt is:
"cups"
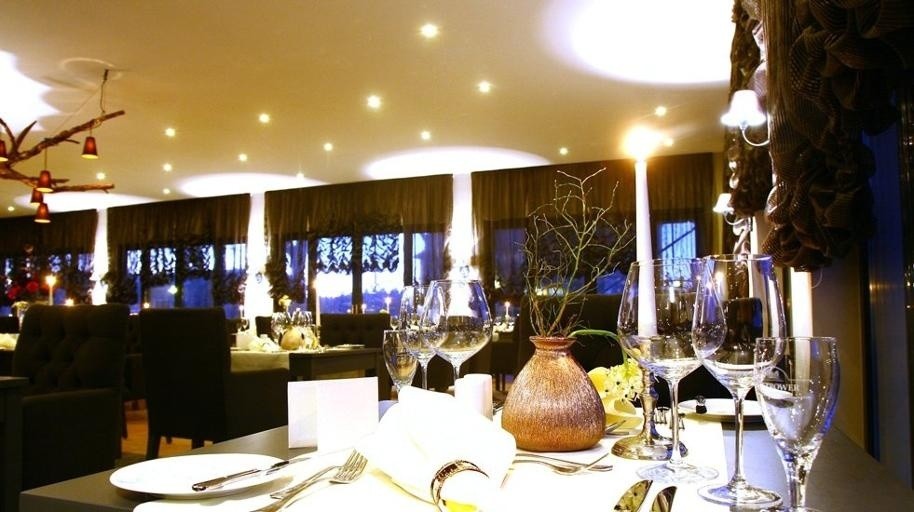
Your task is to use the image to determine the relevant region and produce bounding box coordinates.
[454,372,493,421]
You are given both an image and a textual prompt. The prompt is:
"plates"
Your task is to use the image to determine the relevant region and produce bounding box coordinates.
[110,452,291,497]
[679,396,763,423]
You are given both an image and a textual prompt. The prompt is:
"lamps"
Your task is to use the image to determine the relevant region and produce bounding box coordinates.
[721,89,771,147]
[713,193,746,225]
[0,69,108,223]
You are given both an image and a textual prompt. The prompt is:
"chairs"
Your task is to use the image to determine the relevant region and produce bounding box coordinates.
[0,292,635,512]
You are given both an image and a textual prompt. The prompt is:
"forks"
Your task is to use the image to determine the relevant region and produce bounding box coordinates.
[250,449,368,512]
[512,452,609,476]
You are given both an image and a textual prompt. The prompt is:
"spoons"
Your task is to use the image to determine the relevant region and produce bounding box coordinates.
[517,453,612,472]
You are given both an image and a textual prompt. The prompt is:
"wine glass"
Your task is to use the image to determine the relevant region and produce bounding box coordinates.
[615,260,719,481]
[271,310,315,349]
[693,253,781,507]
[383,280,491,393]
[753,336,839,511]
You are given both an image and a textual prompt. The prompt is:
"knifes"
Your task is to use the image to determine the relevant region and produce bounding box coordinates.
[193,457,309,490]
[614,479,679,512]
